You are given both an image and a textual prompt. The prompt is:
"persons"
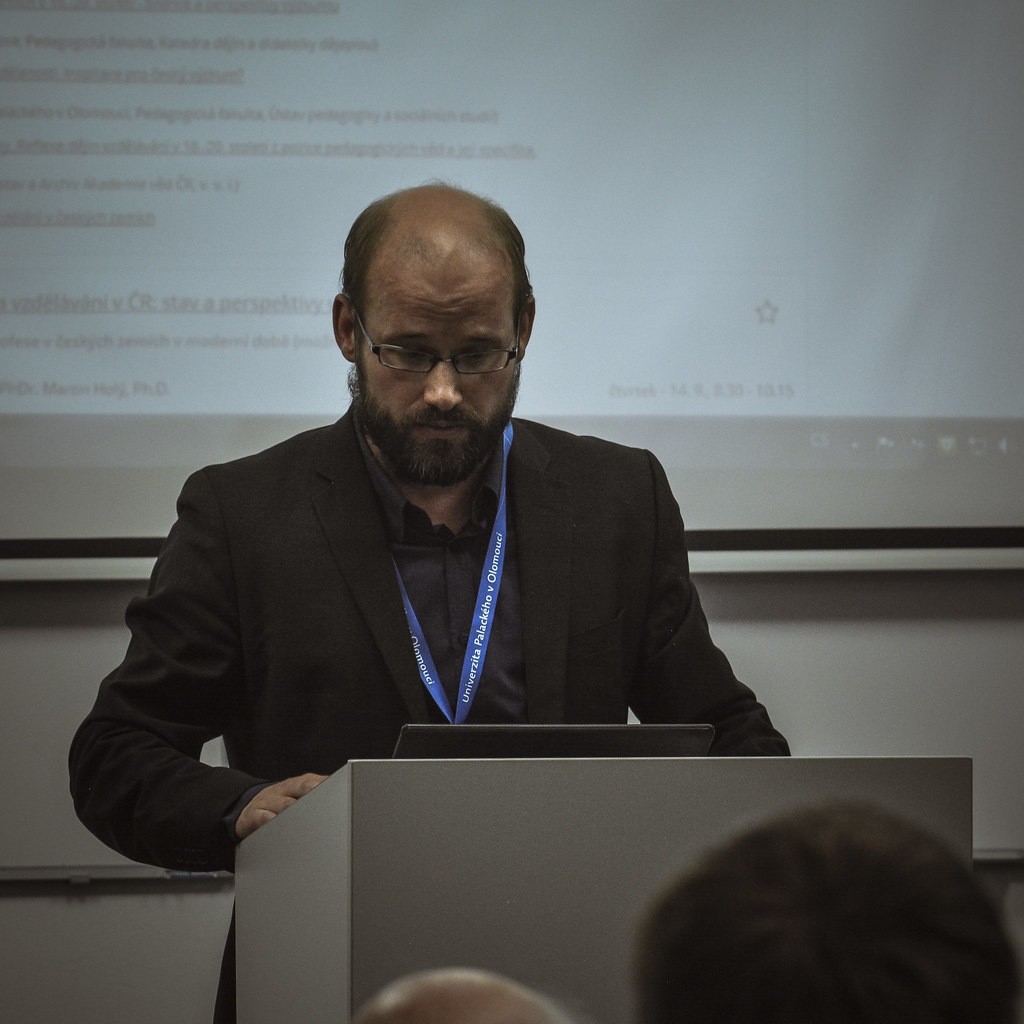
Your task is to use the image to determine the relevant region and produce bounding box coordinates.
[68,185,791,874]
[349,804,1023,1023]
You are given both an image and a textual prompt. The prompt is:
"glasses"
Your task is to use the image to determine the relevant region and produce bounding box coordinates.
[342,291,527,375]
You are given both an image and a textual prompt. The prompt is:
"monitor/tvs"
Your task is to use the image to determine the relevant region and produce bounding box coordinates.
[391,723,716,757]
